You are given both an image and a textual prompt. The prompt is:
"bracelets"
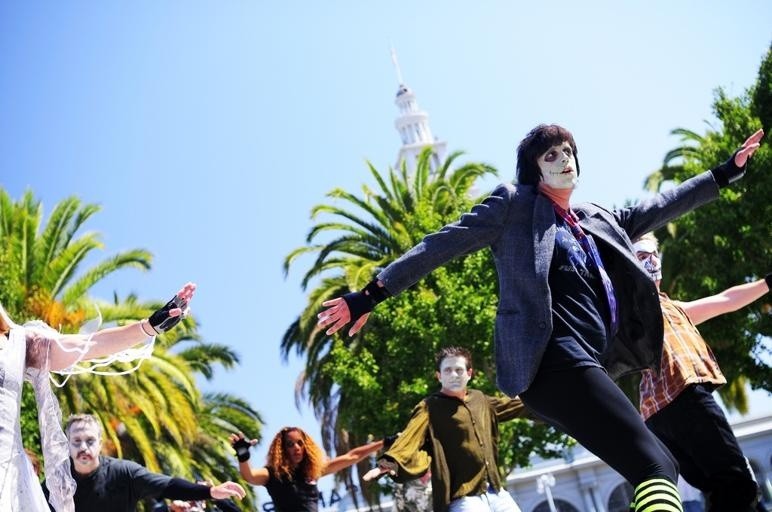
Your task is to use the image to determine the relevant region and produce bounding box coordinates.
[140,319,155,337]
[764,272,772,292]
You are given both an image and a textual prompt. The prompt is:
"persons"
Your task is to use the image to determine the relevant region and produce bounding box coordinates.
[315,122,765,512]
[363,345,527,512]
[1,281,200,512]
[630,233,772,512]
[228,427,403,512]
[40,412,246,512]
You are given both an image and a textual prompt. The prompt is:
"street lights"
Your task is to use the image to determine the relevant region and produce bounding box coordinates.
[537,474,556,512]
